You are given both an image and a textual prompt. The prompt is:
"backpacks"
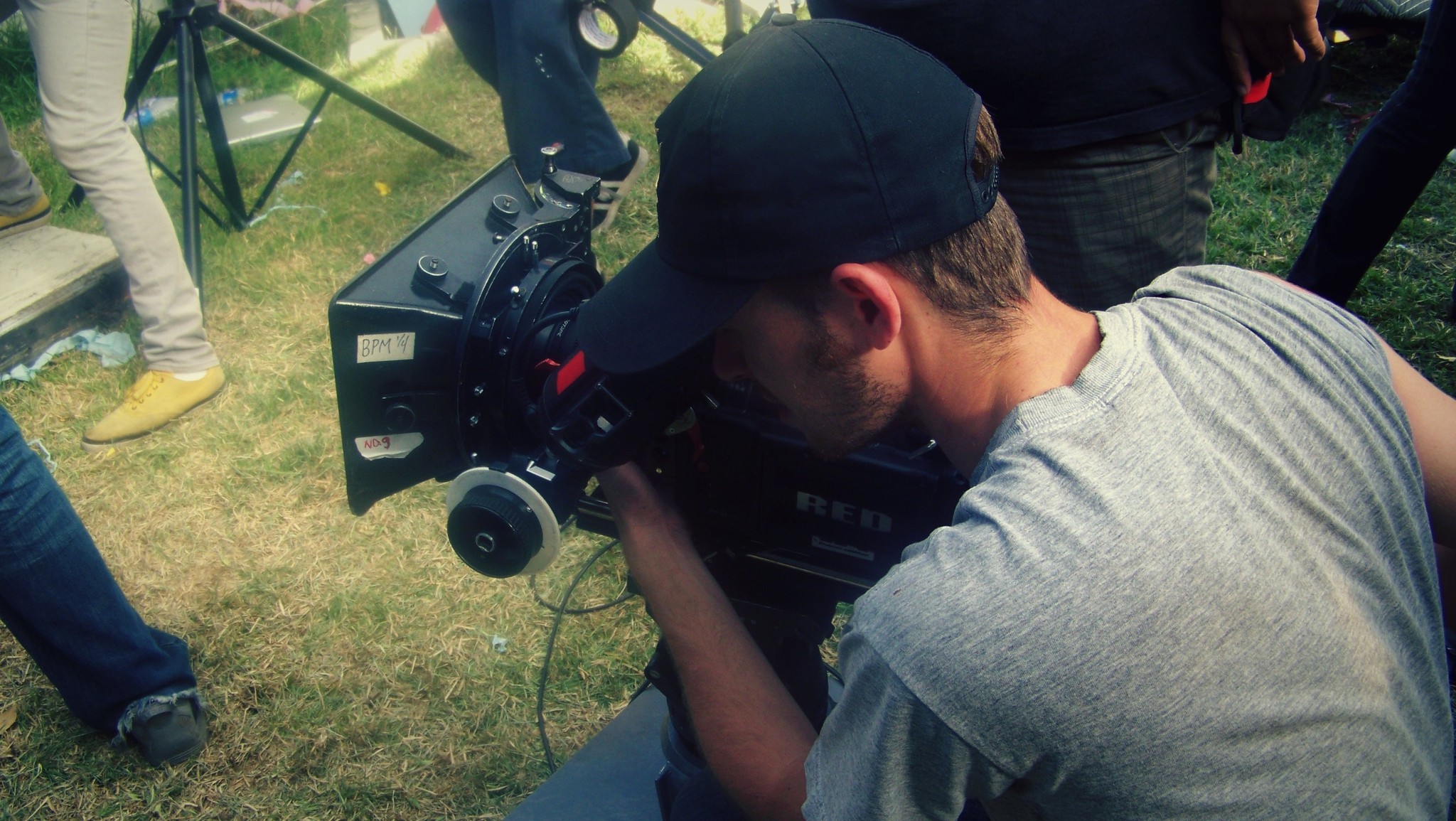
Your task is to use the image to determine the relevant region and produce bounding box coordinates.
[1216,0,1349,154]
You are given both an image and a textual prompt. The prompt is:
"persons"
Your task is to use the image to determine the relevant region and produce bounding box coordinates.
[0,0,230,453]
[436,0,649,239]
[599,13,1455,821]
[0,407,210,771]
[809,1,1329,314]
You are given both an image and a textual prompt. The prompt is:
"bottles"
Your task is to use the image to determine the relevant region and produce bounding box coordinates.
[208,87,263,109]
[126,95,184,131]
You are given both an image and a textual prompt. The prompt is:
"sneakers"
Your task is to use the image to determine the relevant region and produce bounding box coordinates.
[589,133,648,238]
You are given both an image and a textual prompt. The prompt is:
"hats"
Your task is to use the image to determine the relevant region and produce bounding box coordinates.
[572,14,1001,374]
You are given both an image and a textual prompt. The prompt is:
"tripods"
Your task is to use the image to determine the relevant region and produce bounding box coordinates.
[56,0,481,310]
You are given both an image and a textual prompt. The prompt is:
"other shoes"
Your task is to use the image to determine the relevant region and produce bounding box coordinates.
[82,352,224,443]
[131,698,207,767]
[0,189,53,238]
